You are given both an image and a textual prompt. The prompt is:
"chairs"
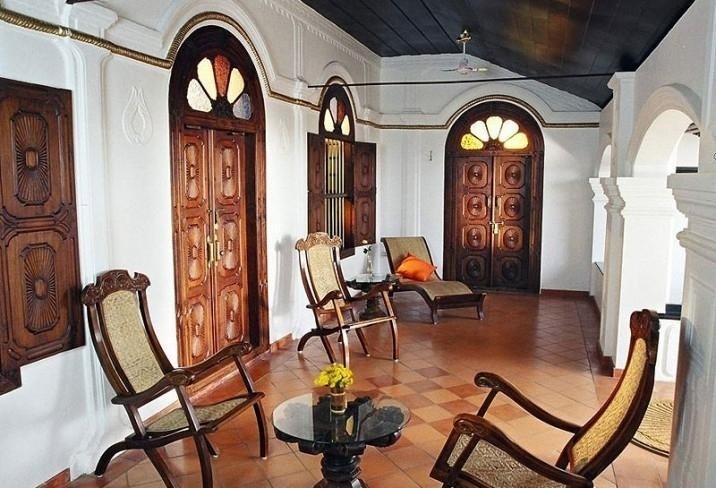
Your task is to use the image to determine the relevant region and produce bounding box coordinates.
[380,236,488,326]
[295,231,400,369]
[81,269,268,488]
[429,308,662,488]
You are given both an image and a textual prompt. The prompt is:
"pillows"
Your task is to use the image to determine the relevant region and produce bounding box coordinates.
[395,252,437,282]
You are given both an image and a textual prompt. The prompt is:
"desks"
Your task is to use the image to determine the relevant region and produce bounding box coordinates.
[271,389,410,488]
[344,273,403,324]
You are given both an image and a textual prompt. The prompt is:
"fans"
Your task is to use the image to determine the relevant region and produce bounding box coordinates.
[440,28,488,75]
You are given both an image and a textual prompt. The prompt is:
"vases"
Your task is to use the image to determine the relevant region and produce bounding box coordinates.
[366,252,375,275]
[330,392,347,414]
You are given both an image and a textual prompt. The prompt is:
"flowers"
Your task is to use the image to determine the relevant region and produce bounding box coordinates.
[360,239,372,254]
[314,363,354,391]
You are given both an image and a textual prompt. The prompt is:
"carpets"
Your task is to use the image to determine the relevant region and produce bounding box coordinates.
[631,397,675,458]
